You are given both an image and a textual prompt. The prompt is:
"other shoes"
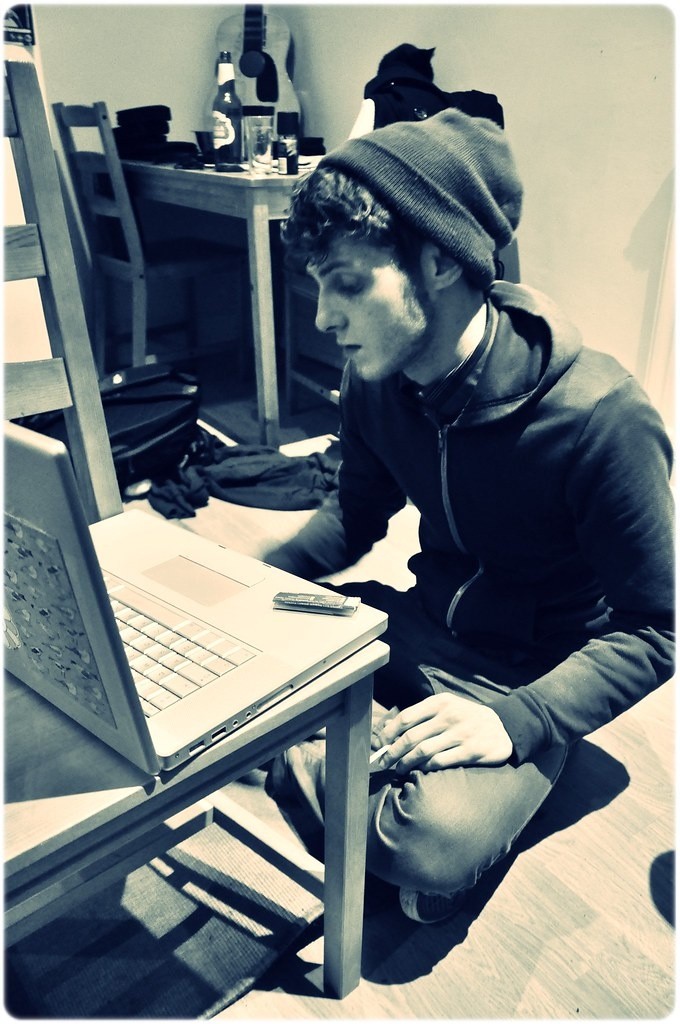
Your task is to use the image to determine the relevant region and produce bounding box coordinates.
[400,885,465,925]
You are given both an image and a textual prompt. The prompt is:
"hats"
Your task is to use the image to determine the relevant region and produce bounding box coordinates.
[318,107,525,288]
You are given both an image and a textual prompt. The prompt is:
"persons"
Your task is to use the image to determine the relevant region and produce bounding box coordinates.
[262,108,675,924]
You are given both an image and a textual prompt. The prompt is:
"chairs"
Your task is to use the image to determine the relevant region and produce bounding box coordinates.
[284,270,359,417]
[53,101,248,374]
[3,61,390,999]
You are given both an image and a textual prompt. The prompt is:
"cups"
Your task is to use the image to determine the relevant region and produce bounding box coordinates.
[242,115,275,176]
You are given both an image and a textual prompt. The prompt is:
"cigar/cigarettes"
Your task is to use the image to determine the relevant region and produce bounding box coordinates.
[369,745,390,764]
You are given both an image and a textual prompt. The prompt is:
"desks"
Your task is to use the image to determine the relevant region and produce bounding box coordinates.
[117,154,332,455]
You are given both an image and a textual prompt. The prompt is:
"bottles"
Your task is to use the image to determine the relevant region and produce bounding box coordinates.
[211,51,243,173]
[277,112,299,175]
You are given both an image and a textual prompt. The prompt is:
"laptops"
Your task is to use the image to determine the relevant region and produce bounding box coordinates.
[3,420,388,775]
